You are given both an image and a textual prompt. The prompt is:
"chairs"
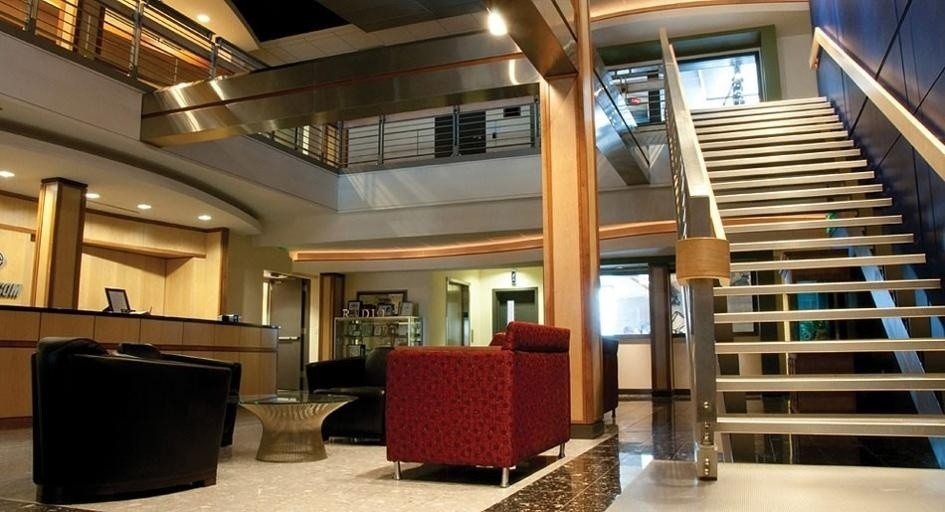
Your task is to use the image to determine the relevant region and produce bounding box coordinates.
[30,336,231,504]
[602,337,619,417]
[383,321,572,489]
[112,342,242,447]
[305,355,387,444]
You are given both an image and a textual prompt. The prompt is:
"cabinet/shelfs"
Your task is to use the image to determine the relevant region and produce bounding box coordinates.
[332,316,423,359]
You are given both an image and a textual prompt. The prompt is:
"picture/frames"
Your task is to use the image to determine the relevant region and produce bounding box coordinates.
[347,300,362,317]
[357,289,408,316]
